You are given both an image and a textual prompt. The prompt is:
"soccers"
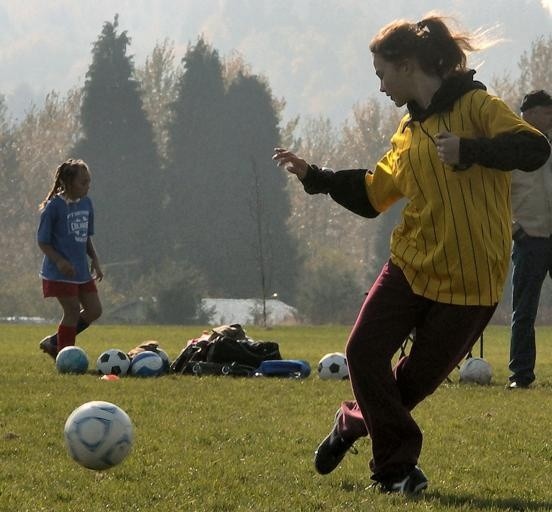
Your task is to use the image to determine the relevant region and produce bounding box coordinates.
[57,346,88,374]
[64,401,133,470]
[316,352,349,380]
[97,349,163,377]
[460,357,492,385]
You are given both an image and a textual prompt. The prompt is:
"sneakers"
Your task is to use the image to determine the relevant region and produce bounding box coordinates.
[507,376,535,389]
[40,337,58,363]
[315,408,357,475]
[371,465,427,497]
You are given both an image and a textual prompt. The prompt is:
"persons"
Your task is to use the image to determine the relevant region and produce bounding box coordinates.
[505,88,552,389]
[271,11,551,494]
[37,159,104,358]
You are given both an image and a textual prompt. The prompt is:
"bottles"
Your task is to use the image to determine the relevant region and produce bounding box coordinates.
[102,374,119,381]
